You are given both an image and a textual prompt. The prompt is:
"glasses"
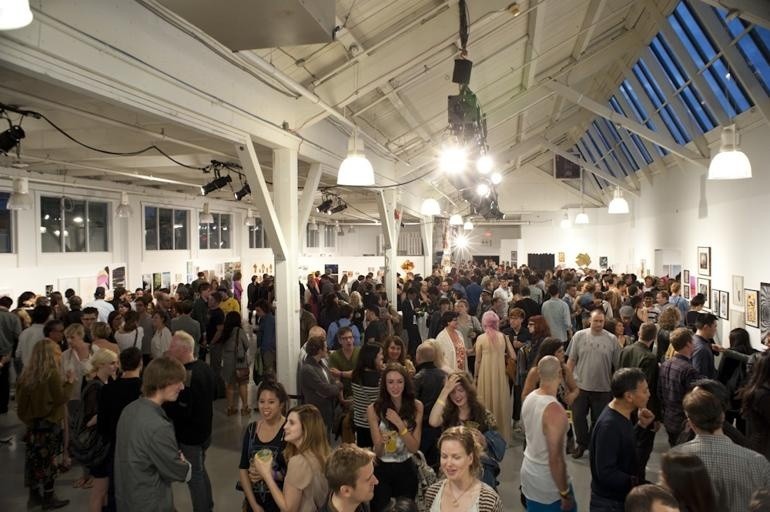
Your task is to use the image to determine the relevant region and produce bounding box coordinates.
[528,323,534,326]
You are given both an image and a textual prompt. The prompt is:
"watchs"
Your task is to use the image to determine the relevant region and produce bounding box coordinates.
[558,488,570,497]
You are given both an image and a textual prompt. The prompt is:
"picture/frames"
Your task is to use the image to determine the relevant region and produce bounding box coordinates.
[684,270,689,284]
[719,290,729,320]
[743,289,759,328]
[684,285,689,299]
[697,246,711,276]
[760,282,770,347]
[696,277,710,310]
[731,275,744,307]
[710,289,719,316]
[729,309,744,331]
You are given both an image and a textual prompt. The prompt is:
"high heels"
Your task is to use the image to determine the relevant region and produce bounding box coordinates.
[227,407,238,416]
[241,406,251,416]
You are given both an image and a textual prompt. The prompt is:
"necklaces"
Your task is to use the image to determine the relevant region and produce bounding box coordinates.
[448,480,475,507]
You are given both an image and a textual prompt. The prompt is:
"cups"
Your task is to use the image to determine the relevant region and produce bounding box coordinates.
[249,459,255,468]
[256,448,272,462]
[385,432,398,453]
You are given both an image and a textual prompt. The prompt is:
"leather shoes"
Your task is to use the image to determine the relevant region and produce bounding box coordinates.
[27,495,42,510]
[571,445,588,458]
[41,496,70,511]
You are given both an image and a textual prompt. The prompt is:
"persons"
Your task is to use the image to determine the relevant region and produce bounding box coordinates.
[664,324,720,363]
[368,363,424,512]
[383,335,415,375]
[625,483,680,512]
[472,310,517,447]
[0,268,241,512]
[318,442,419,512]
[351,341,384,449]
[413,339,450,476]
[669,283,689,327]
[435,311,475,374]
[301,325,333,385]
[521,338,579,455]
[502,307,531,432]
[719,328,762,436]
[659,328,701,446]
[643,291,661,323]
[748,487,770,512]
[721,335,770,373]
[656,290,671,312]
[239,380,290,512]
[241,269,403,343]
[604,319,633,347]
[423,424,503,511]
[670,386,770,512]
[734,353,770,447]
[616,322,660,483]
[685,293,705,332]
[519,356,579,512]
[327,327,361,443]
[564,308,624,459]
[428,372,506,493]
[589,369,655,512]
[658,450,715,512]
[404,259,629,310]
[617,306,640,342]
[675,381,757,451]
[631,296,647,342]
[631,273,680,291]
[524,316,552,371]
[657,305,681,365]
[299,336,342,445]
[255,403,334,512]
[686,314,719,379]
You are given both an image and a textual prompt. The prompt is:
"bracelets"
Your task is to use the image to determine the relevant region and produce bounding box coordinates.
[400,428,408,436]
[435,397,446,407]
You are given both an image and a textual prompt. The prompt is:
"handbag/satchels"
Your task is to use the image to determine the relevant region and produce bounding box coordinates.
[67,381,103,460]
[234,328,250,382]
[504,336,516,385]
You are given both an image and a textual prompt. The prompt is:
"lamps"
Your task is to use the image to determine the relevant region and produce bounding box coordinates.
[0,0,34,31]
[705,128,754,181]
[0,115,26,156]
[464,222,473,230]
[449,215,464,226]
[328,200,347,215]
[234,184,251,201]
[200,169,232,196]
[419,198,440,216]
[607,188,629,215]
[335,61,376,187]
[575,211,588,225]
[316,195,332,214]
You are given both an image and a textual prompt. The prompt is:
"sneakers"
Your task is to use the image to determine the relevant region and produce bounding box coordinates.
[513,421,522,432]
[566,437,575,454]
